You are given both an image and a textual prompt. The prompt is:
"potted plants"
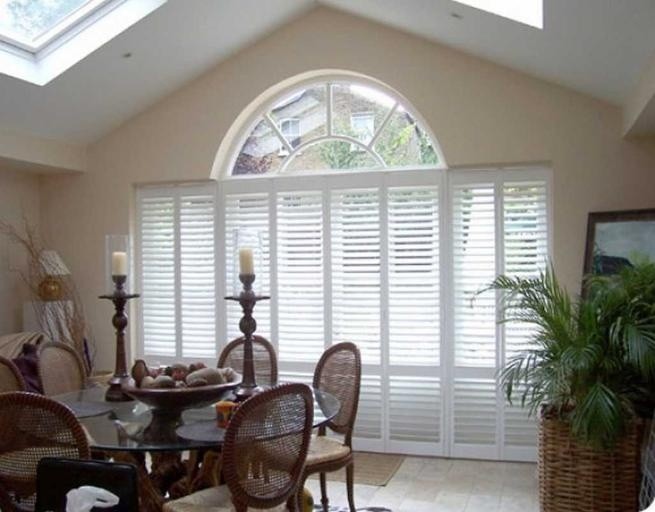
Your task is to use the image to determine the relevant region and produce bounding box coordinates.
[468,257,653,511]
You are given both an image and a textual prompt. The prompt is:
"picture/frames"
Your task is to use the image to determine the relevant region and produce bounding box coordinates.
[582,207,655,317]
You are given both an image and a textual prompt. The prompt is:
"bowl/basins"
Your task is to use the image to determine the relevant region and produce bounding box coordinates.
[121,377,243,408]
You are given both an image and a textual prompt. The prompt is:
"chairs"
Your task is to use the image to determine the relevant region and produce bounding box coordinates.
[0,391,92,512]
[35,339,90,394]
[242,338,370,512]
[216,334,277,384]
[36,457,137,512]
[0,355,28,392]
[159,379,312,512]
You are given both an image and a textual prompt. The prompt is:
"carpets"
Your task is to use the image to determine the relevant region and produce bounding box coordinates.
[307,450,406,487]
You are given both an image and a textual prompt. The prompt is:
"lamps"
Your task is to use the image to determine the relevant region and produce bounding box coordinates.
[31,240,72,302]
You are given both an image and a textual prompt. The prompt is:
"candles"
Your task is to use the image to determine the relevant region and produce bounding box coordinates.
[112,250,128,277]
[239,247,255,276]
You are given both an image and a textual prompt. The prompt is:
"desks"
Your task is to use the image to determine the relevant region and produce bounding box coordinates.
[41,372,340,512]
